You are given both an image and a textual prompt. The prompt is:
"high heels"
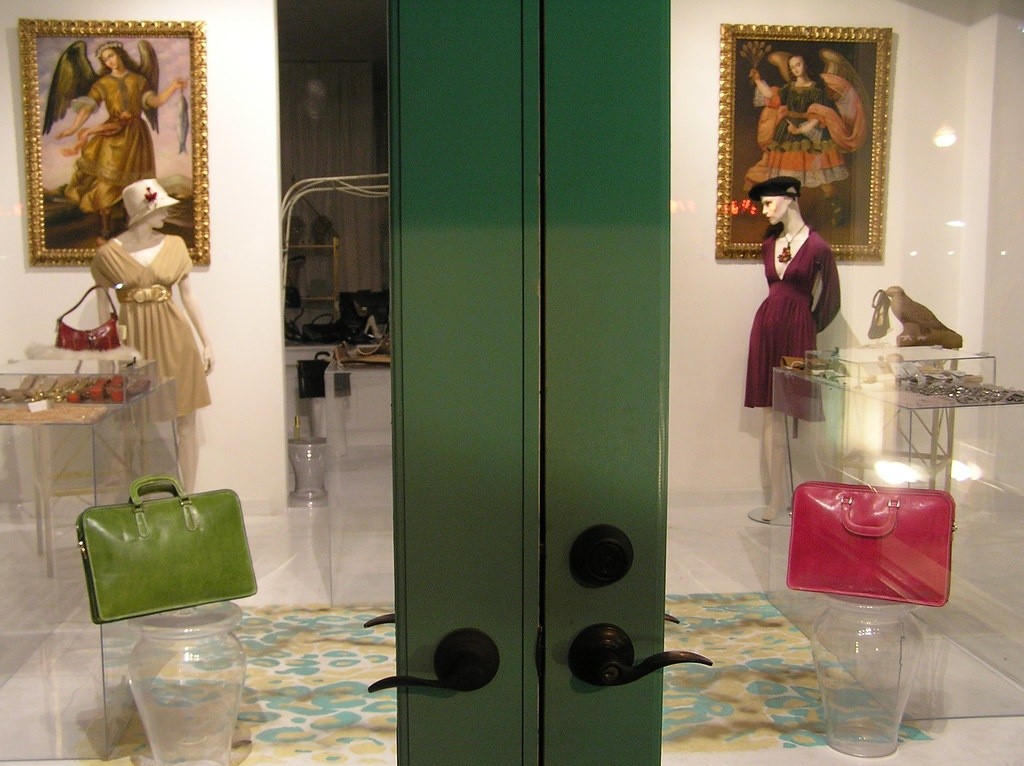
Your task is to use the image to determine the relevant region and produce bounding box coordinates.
[364,315,382,339]
[868,290,892,339]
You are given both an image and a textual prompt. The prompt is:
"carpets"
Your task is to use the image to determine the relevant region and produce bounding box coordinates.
[106,588,934,766]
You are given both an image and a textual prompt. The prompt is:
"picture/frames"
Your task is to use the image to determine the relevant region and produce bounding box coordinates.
[18,18,211,268]
[715,23,892,265]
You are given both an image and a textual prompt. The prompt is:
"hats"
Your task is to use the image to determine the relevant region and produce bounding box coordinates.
[122,178,180,227]
[748,176,801,201]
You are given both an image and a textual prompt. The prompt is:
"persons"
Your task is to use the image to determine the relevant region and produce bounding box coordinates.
[90,179,215,492]
[743,177,840,521]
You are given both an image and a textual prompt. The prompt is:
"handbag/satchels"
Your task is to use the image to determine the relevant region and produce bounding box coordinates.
[301,313,335,343]
[286,278,299,307]
[790,481,958,607]
[75,476,259,624]
[57,285,122,350]
[298,351,332,398]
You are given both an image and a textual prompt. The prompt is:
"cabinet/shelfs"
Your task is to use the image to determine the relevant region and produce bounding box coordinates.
[286,236,340,324]
[286,345,391,447]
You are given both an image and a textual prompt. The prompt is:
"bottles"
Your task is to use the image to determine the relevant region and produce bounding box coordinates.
[811,593,925,757]
[288,438,330,499]
[126,601,247,766]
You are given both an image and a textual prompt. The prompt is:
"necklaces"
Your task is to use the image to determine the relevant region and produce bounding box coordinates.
[777,222,806,263]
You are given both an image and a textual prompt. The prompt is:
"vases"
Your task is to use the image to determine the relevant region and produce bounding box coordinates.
[288,438,328,499]
